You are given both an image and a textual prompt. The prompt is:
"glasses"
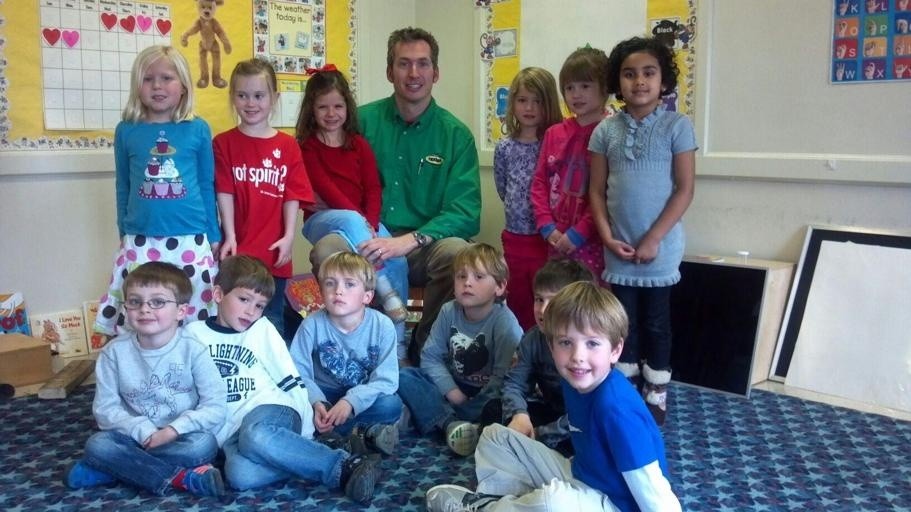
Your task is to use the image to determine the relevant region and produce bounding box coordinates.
[123,298,184,310]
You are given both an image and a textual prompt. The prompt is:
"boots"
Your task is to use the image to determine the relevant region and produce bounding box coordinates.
[641,362,672,427]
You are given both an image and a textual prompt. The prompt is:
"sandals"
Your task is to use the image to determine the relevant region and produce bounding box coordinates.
[377,289,409,325]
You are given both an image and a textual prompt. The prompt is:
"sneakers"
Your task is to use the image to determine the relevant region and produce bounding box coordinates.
[314,422,402,510]
[425,483,504,512]
[184,464,226,500]
[445,421,482,456]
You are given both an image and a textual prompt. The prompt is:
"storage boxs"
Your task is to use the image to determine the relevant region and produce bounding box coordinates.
[0,331,54,391]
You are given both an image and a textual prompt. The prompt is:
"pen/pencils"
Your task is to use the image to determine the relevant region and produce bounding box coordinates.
[416,157,424,175]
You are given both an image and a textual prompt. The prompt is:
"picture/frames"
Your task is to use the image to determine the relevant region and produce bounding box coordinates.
[762,224,908,386]
[26,307,88,361]
[666,259,769,402]
[80,299,116,353]
